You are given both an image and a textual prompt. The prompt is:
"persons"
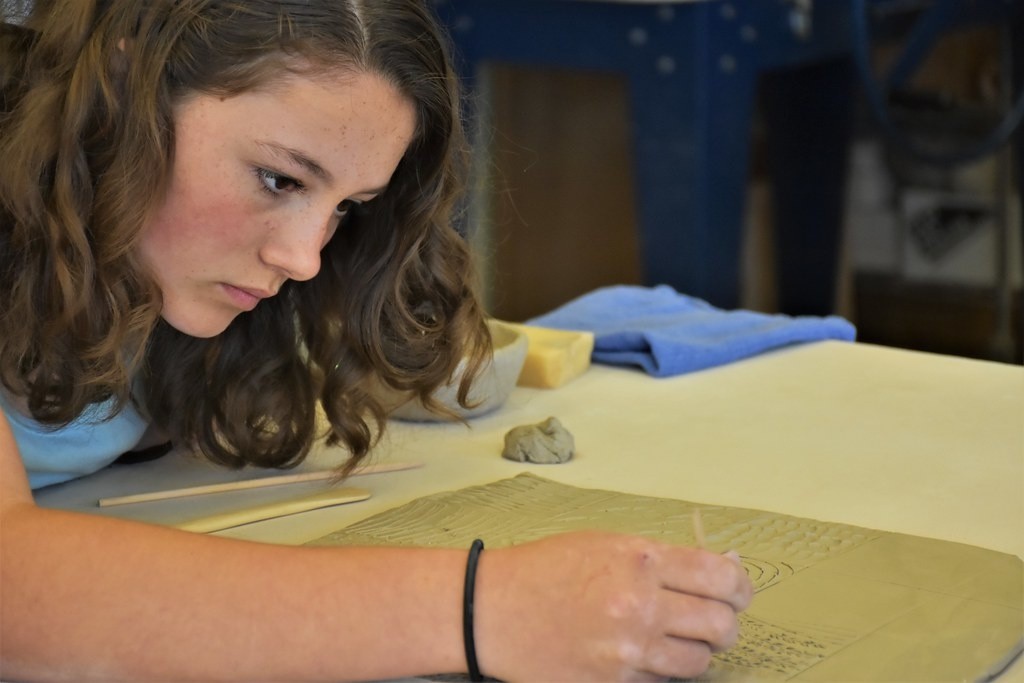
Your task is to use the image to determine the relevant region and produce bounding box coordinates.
[0,0,755,683]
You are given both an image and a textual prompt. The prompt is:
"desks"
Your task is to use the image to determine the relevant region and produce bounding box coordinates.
[30,330,1023,682]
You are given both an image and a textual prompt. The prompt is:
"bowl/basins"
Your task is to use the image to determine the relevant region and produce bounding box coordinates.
[360,322,528,422]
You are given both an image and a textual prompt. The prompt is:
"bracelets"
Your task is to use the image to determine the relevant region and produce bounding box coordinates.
[463,539,485,679]
